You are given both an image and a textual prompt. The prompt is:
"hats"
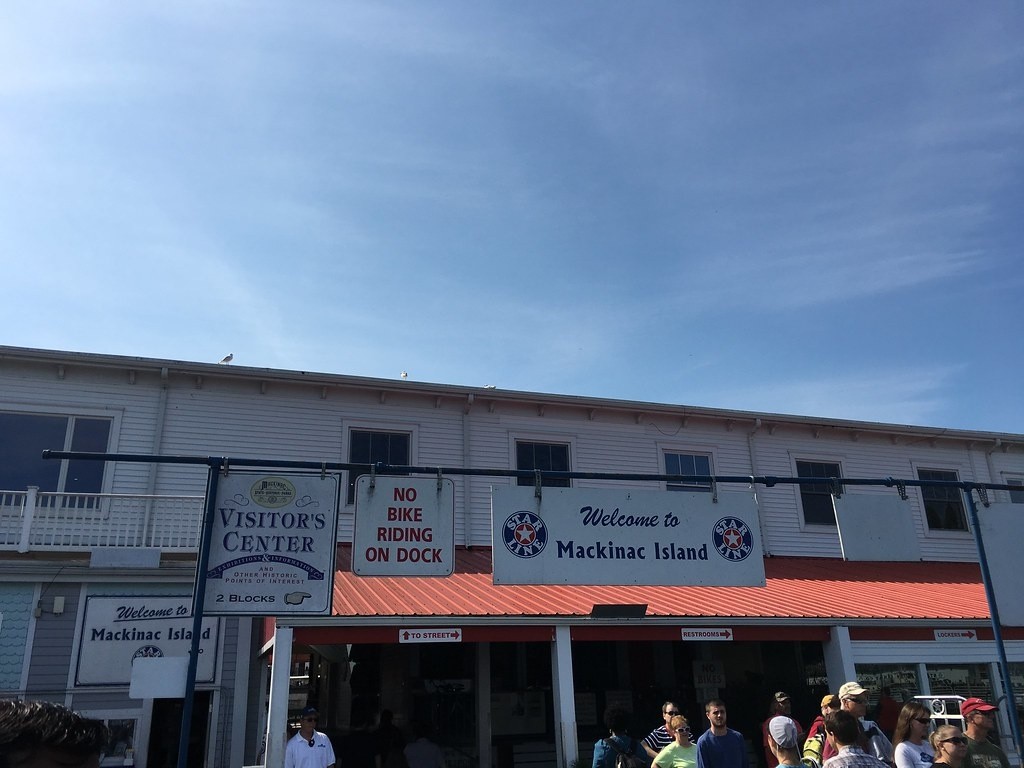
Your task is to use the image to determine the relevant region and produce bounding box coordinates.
[961,697,1000,717]
[821,694,840,707]
[770,715,798,748]
[298,706,320,715]
[838,682,870,699]
[764,686,791,712]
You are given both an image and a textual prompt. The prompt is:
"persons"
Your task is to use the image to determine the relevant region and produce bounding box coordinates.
[873,685,911,743]
[823,682,869,766]
[592,704,647,768]
[336,709,446,768]
[640,701,696,758]
[823,710,890,768]
[929,725,968,768]
[804,695,841,766]
[651,715,697,768]
[697,698,746,768]
[287,707,337,768]
[894,702,936,768]
[766,715,809,768]
[961,698,1010,768]
[762,687,803,768]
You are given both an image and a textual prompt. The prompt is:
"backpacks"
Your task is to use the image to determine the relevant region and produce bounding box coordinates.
[604,736,647,768]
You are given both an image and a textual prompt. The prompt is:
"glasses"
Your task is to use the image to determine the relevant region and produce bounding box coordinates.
[301,718,319,722]
[675,727,691,733]
[941,736,968,745]
[914,718,932,725]
[666,711,682,716]
[847,698,868,706]
[969,710,995,717]
